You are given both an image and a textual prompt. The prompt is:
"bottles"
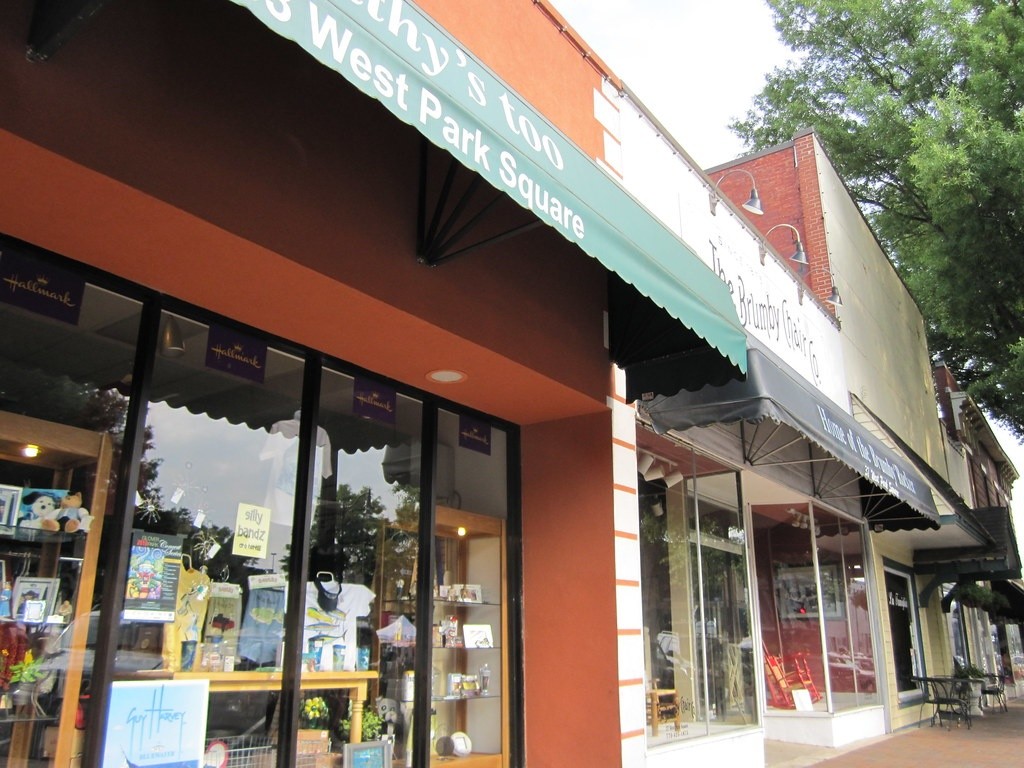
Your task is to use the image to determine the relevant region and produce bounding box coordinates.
[402,670,415,701]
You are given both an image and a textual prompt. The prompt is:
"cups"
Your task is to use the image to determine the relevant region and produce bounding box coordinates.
[181,640,197,671]
[333,645,346,671]
[356,645,370,670]
[310,638,324,671]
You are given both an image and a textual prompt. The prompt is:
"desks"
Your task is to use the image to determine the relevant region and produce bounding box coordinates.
[913,677,984,727]
[116,669,378,751]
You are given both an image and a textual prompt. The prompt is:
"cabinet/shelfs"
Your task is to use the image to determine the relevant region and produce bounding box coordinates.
[0,411,509,768]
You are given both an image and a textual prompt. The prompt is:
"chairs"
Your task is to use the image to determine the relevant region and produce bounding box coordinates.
[911,655,1008,731]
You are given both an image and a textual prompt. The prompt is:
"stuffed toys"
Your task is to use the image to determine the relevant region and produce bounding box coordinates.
[38,492,91,534]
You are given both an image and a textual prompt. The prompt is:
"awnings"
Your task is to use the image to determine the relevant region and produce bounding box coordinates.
[645,324,942,532]
[27,2,751,404]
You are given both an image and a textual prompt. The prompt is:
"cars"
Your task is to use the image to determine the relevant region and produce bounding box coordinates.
[828,652,860,690]
[850,652,876,690]
[11,602,165,727]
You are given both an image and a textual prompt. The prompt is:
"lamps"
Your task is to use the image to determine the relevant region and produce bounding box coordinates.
[787,508,820,536]
[651,496,663,517]
[710,169,763,216]
[637,449,682,488]
[797,268,843,307]
[760,224,808,267]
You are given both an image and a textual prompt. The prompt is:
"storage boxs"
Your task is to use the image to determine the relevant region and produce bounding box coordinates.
[297,728,328,751]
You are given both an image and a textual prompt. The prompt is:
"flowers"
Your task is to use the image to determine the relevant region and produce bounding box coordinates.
[299,697,329,727]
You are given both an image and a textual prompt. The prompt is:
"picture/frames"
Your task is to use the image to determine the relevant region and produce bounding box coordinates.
[25,601,45,623]
[451,584,482,603]
[462,624,494,648]
[344,741,394,768]
[12,577,60,619]
[772,562,845,621]
[0,484,22,533]
[882,555,930,709]
